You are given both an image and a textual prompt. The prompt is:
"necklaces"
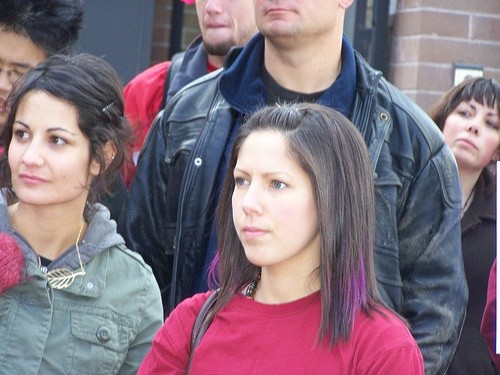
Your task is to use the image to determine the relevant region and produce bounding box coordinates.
[463,186,474,211]
[246,276,259,300]
[12,202,86,290]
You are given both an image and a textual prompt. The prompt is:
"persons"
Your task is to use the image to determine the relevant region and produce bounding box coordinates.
[0,0,259,217]
[116,0,469,375]
[0,53,164,375]
[135,102,426,375]
[428,77,500,375]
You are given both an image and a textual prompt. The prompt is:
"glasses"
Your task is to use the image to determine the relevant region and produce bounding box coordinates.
[0,67,24,84]
